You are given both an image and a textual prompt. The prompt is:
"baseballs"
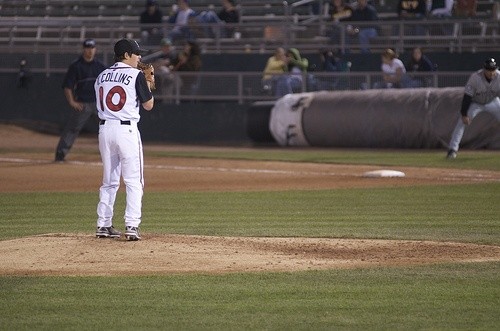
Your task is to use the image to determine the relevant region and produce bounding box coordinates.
[449,151,457,162]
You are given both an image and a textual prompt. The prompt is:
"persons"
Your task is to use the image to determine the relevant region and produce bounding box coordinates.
[446,58,500,160]
[94,39,156,240]
[130,0,500,101]
[56,39,109,161]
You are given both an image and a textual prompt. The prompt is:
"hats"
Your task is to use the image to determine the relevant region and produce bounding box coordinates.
[83,39,97,48]
[483,57,497,71]
[114,40,150,54]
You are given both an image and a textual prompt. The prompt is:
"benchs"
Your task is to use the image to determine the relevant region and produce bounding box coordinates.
[1,0,500,101]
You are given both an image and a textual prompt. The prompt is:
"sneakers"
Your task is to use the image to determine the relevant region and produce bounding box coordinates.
[95,226,121,238]
[55,157,65,162]
[446,149,457,160]
[125,226,142,241]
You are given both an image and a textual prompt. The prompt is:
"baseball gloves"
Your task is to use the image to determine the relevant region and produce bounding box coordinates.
[138,62,156,90]
